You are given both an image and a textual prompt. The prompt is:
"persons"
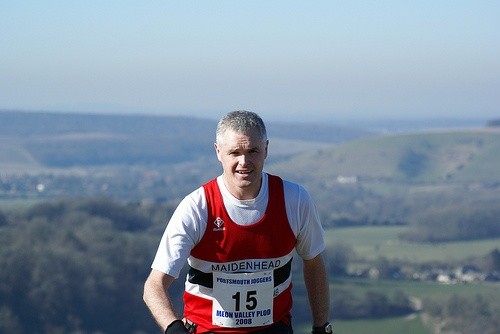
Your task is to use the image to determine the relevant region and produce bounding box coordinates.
[141,110,333,334]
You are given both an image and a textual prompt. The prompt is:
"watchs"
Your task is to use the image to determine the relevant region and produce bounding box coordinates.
[312,323,333,334]
